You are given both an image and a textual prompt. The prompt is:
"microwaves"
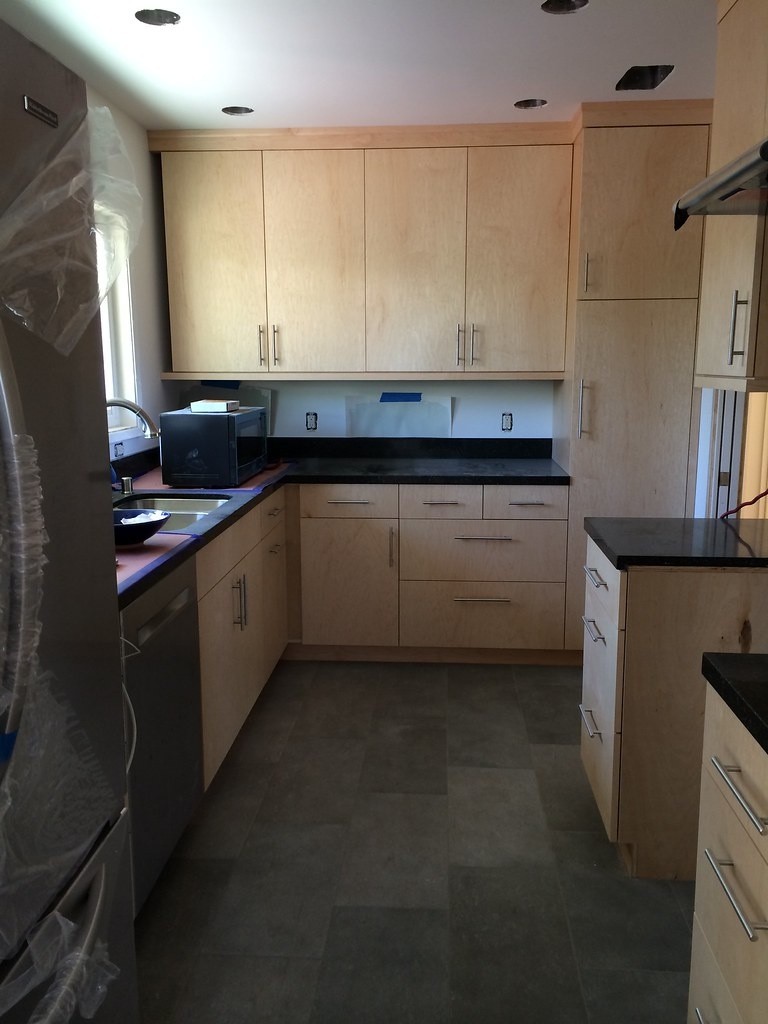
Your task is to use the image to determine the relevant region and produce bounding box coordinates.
[159,405,266,490]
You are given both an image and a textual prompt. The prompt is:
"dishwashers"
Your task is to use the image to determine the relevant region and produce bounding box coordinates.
[119,552,206,916]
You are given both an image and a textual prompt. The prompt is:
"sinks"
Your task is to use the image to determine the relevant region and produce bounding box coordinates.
[113,492,234,512]
[159,512,207,531]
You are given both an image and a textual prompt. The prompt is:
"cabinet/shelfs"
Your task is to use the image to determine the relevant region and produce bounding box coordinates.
[686,654,768,1024]
[364,122,573,380]
[579,517,768,880]
[196,504,264,794]
[286,483,400,661]
[553,99,700,668]
[399,484,569,666]
[145,127,366,380]
[260,484,288,682]
[692,1,767,393]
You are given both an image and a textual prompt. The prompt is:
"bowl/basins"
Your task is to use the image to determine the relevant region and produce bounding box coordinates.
[113,508,171,548]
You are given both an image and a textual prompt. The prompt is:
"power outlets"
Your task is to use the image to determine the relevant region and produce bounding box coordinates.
[503,415,511,429]
[307,415,316,428]
[115,444,124,457]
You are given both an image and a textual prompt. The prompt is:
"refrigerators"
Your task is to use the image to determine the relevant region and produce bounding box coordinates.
[1,20,141,1024]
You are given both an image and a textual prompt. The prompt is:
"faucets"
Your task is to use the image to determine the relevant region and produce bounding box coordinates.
[106,398,162,439]
